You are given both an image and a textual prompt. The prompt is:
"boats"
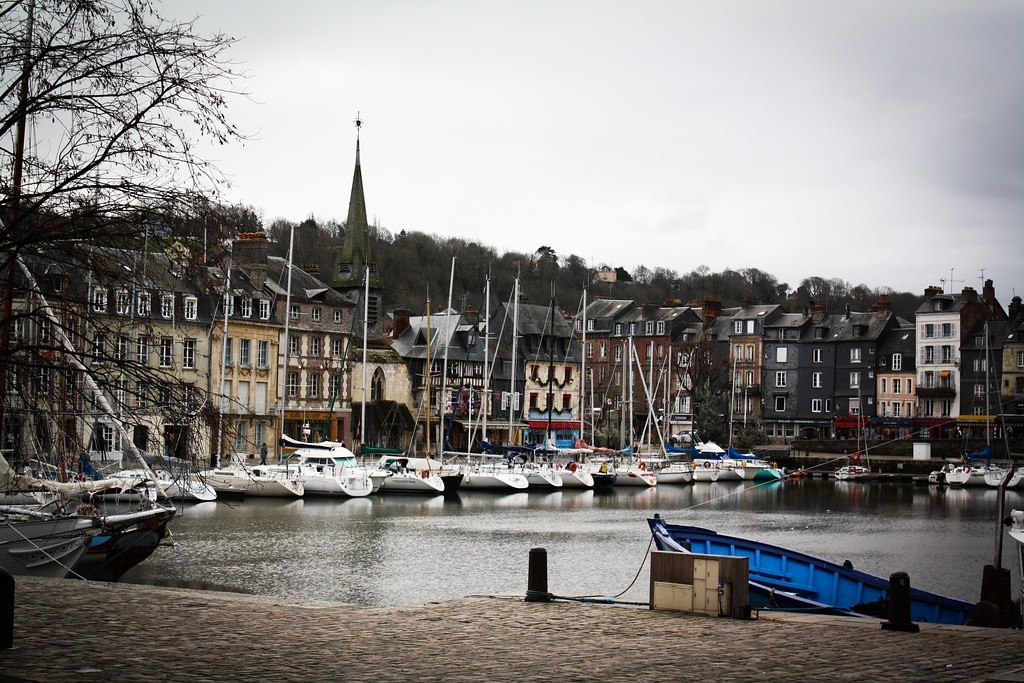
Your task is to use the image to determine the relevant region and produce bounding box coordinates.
[0,497,106,580]
[835,466,869,479]
[928,462,1024,488]
[646,513,974,627]
[104,470,218,502]
[279,432,393,493]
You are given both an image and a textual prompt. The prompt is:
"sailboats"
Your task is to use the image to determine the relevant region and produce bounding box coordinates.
[319,255,783,495]
[172,268,305,498]
[231,226,374,498]
[0,219,178,583]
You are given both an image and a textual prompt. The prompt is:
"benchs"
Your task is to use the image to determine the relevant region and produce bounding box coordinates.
[748,572,819,592]
[748,564,793,578]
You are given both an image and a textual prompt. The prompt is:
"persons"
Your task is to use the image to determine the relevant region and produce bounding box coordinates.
[260,442,268,465]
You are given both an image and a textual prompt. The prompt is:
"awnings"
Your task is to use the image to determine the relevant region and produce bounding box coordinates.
[939,370,950,377]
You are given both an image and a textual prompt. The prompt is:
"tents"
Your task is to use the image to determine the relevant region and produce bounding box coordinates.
[484,439,615,466]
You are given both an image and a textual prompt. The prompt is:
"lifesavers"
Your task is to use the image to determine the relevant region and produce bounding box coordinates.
[963,465,971,473]
[569,463,577,472]
[421,470,430,479]
[639,462,645,470]
[703,461,711,468]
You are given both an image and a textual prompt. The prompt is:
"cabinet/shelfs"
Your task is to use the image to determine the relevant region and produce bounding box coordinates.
[648,550,749,619]
